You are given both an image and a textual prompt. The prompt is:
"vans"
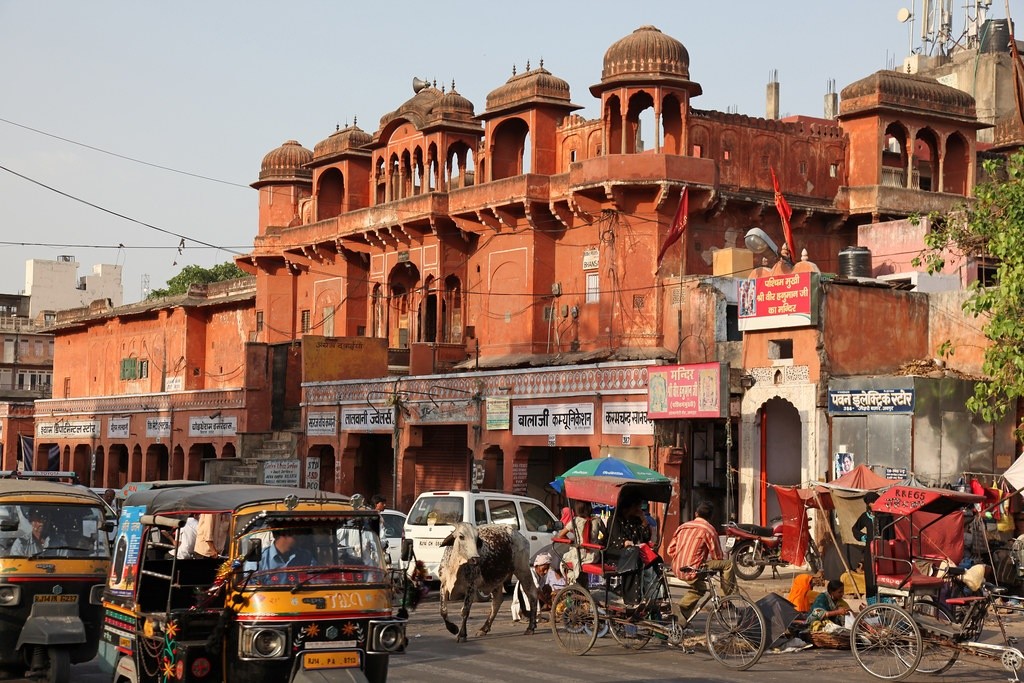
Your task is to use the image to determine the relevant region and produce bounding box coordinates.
[0,488,118,548]
[402,488,567,603]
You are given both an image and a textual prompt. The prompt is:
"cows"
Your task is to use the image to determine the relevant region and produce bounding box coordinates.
[438,519,539,643]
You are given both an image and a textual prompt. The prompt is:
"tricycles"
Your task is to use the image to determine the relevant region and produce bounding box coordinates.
[548,456,768,672]
[849,488,1024,683]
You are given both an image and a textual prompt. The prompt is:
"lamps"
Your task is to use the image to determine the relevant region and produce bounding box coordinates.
[739,375,753,388]
[743,227,795,267]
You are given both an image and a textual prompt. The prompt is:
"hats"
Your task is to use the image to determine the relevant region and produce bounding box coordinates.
[534,553,552,566]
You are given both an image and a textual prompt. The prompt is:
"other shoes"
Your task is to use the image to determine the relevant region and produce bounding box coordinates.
[718,600,739,613]
[679,604,691,620]
[624,603,641,609]
[536,614,548,623]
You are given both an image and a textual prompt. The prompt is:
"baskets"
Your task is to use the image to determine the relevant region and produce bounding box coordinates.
[808,631,852,649]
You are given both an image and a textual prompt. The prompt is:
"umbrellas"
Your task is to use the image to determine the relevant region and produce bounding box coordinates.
[549,454,670,517]
[736,592,802,652]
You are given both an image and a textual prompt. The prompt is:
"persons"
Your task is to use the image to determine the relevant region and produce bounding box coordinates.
[247,521,331,584]
[787,574,855,632]
[852,492,896,612]
[667,501,741,630]
[143,513,231,559]
[842,453,853,472]
[511,499,671,621]
[10,507,73,557]
[336,493,397,600]
[104,489,121,516]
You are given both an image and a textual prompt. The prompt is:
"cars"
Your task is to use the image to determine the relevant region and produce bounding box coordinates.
[326,509,409,580]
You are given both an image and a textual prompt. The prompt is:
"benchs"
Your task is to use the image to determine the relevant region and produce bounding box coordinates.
[871,539,950,592]
[946,596,986,604]
[137,558,228,635]
[580,521,658,575]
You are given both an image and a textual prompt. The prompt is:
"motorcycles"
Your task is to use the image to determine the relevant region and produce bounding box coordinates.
[95,485,410,683]
[722,506,824,581]
[0,477,119,683]
